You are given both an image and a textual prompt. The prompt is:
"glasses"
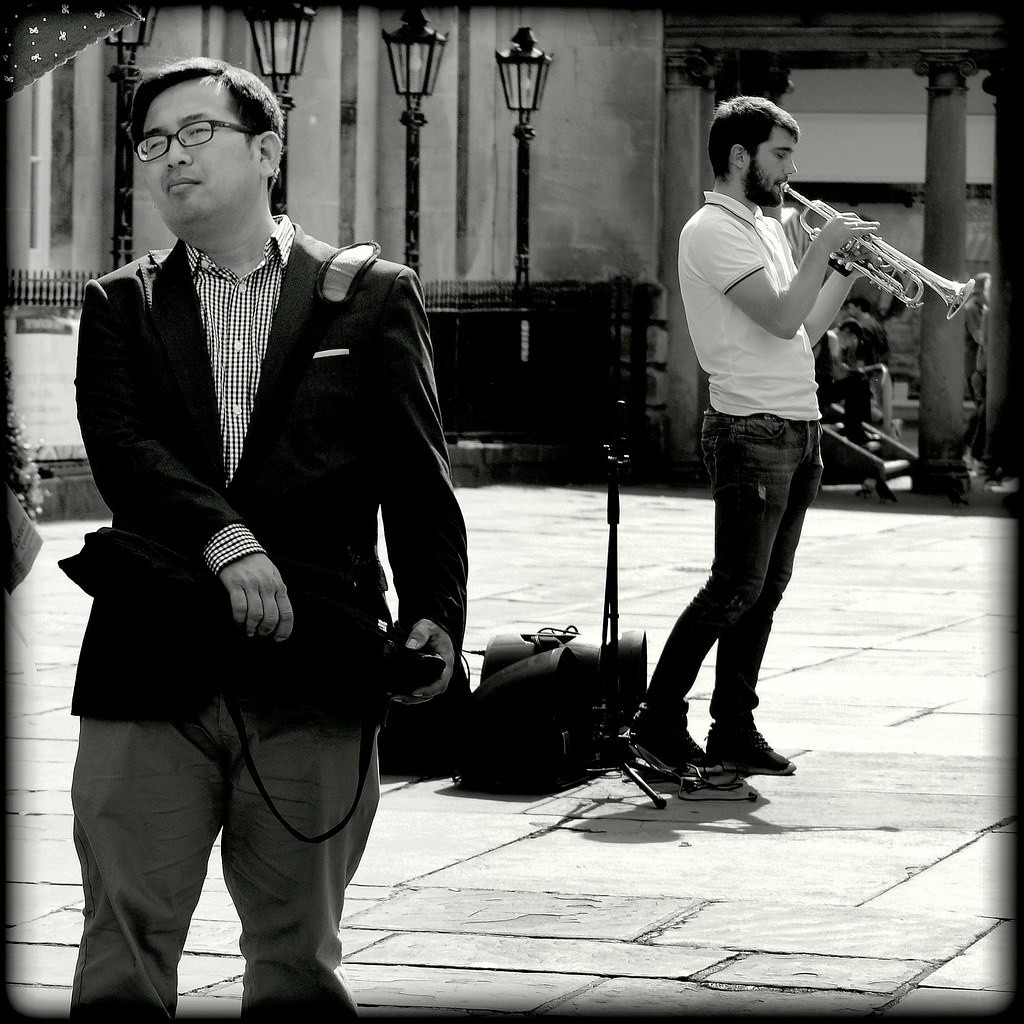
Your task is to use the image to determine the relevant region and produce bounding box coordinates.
[132,119,253,164]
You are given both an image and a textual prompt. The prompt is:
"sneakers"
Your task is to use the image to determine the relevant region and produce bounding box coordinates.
[705,719,796,776]
[630,703,725,774]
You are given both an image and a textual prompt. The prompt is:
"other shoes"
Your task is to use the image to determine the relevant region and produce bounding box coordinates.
[963,455,987,475]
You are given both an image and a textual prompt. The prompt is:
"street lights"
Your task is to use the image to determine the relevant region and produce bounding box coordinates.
[381,6,450,277]
[494,27,554,308]
[105,8,162,272]
[241,2,317,215]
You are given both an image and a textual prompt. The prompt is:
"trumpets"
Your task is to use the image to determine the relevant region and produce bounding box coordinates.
[775,181,975,321]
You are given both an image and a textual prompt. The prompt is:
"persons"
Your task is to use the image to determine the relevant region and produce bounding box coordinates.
[964,273,994,476]
[812,296,897,452]
[631,96,881,776]
[57,56,470,1023]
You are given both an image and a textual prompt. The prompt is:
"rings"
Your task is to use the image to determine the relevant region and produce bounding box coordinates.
[854,222,858,227]
